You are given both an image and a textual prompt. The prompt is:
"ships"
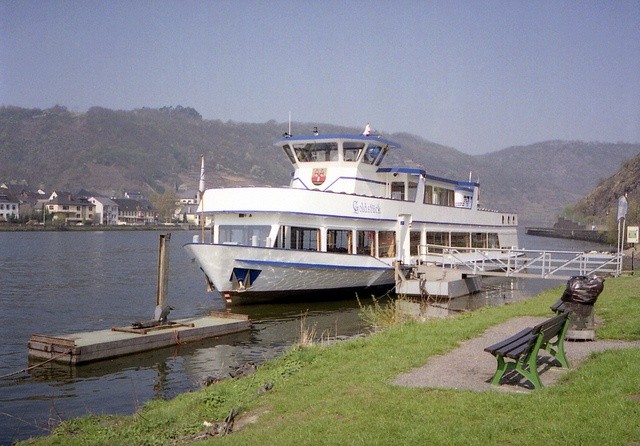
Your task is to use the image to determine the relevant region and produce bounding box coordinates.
[183,111,519,306]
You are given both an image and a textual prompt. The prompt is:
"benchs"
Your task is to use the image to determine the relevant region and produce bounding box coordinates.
[483,311,570,390]
